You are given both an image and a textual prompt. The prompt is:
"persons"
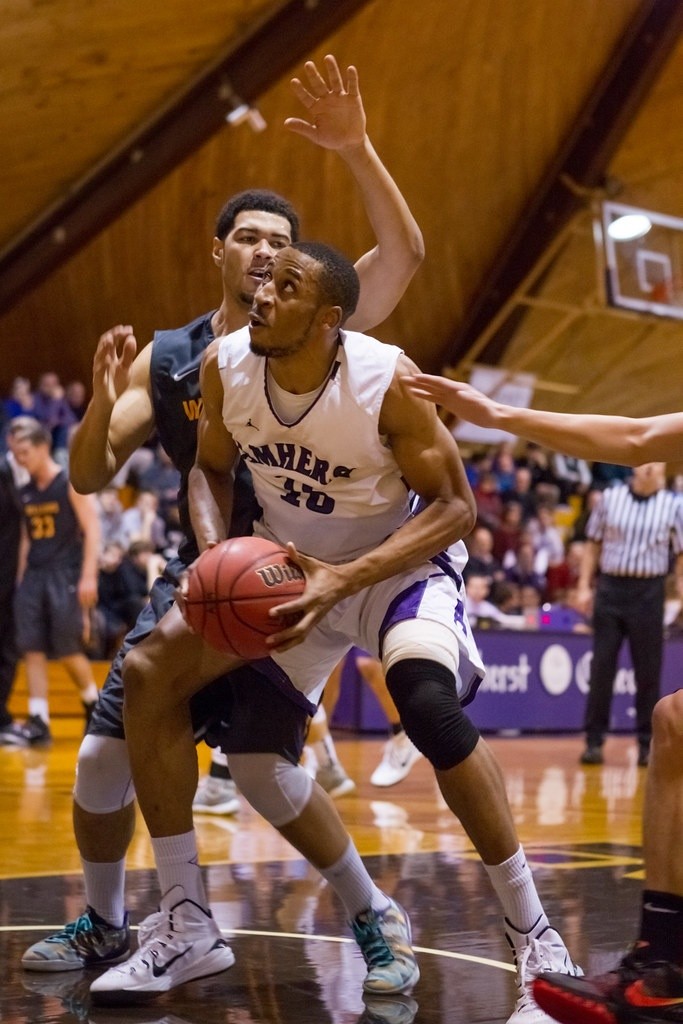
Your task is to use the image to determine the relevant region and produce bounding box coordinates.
[0,52,683,1024]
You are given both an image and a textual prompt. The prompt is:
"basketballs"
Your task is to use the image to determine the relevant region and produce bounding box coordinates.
[180,536,311,656]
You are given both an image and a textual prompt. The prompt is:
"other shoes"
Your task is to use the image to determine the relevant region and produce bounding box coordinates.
[578,746,604,763]
[637,748,650,768]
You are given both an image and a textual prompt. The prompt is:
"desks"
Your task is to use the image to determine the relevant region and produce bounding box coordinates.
[333,629,683,736]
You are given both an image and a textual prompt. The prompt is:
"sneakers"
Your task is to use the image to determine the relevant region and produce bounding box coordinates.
[192,775,239,814]
[0,723,30,747]
[90,885,235,1004]
[370,730,423,787]
[344,890,421,994]
[502,915,587,1024]
[22,713,51,745]
[21,905,131,972]
[535,940,683,1023]
[20,968,98,1021]
[361,994,418,1024]
[315,765,356,798]
[80,698,97,734]
